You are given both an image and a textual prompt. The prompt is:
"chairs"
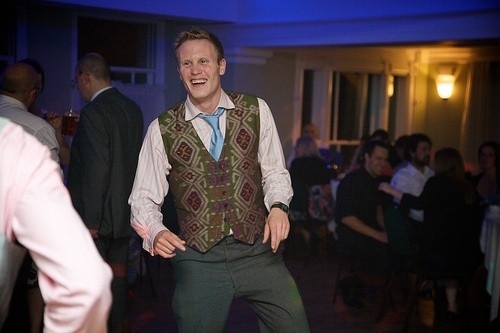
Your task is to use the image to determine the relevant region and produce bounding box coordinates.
[287,185,334,265]
[333,189,461,333]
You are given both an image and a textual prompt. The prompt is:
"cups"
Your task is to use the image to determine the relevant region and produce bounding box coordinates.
[62,104,81,136]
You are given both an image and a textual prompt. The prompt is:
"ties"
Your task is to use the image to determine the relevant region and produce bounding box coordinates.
[198,107,225,162]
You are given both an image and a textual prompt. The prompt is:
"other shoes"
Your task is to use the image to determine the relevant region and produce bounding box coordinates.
[418,287,432,298]
[447,310,459,323]
[344,295,363,308]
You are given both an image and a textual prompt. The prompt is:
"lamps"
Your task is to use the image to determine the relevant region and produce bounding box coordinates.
[436,74,455,100]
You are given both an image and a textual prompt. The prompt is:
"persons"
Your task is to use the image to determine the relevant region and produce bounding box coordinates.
[128,27,309,333]
[0,51,144,333]
[286,121,500,333]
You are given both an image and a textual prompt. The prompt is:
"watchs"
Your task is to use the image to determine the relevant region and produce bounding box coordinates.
[270,204,290,214]
